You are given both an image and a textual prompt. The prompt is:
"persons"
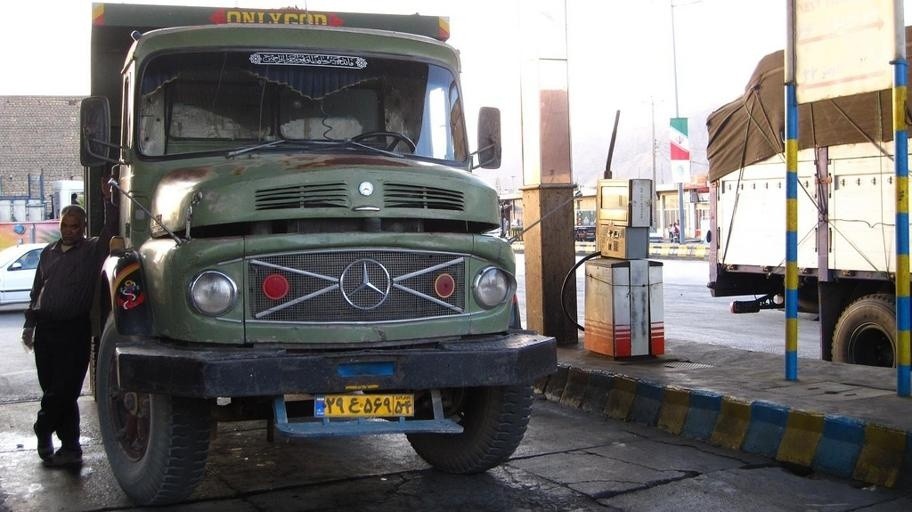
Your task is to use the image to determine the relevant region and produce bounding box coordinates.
[19,172,116,470]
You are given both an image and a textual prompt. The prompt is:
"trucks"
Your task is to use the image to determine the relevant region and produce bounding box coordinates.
[0,176,84,224]
[704,24,912,370]
[78,0,566,507]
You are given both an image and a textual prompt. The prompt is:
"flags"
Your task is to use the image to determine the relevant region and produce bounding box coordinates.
[667,117,692,183]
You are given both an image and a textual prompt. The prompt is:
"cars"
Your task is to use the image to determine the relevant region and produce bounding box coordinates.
[0,242,52,313]
[648,225,664,243]
[511,219,523,226]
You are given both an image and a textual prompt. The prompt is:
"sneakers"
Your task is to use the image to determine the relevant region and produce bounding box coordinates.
[42,449,83,468]
[33,422,54,461]
